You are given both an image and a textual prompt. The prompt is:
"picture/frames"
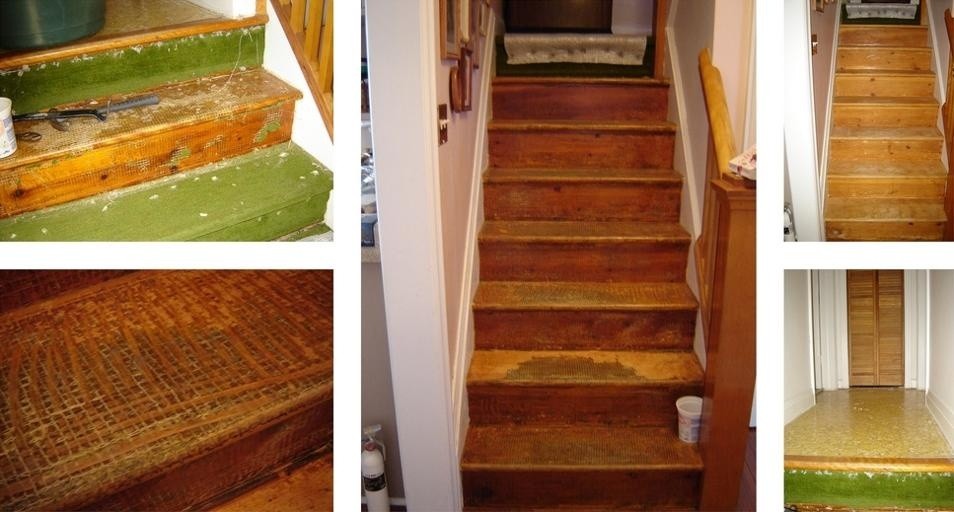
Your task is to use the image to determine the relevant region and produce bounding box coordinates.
[432,1,493,143]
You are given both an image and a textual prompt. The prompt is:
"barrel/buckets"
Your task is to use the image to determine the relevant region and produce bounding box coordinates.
[0,97,18,158]
[676,395,703,443]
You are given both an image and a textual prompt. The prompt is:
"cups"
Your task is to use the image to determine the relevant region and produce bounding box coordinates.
[674,395,702,442]
[0,97,17,159]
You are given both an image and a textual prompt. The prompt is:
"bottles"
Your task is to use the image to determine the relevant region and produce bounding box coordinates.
[361,442,386,512]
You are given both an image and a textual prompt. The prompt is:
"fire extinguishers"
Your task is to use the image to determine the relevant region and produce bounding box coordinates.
[361,425,391,512]
